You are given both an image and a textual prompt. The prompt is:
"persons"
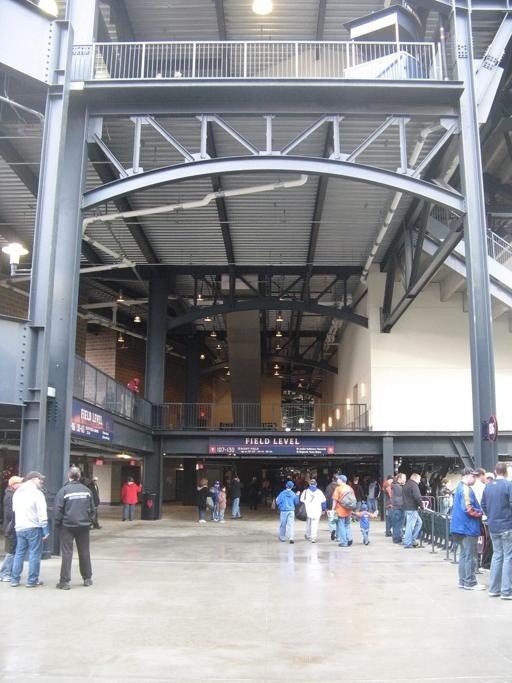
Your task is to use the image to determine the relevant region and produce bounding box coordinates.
[102,384,119,412]
[8,469,51,588]
[470,463,512,599]
[120,374,142,421]
[118,475,143,522]
[0,474,25,582]
[383,472,425,548]
[301,478,325,543]
[440,479,456,514]
[52,465,96,591]
[275,480,300,544]
[197,476,271,523]
[87,475,103,532]
[450,466,487,591]
[326,473,382,548]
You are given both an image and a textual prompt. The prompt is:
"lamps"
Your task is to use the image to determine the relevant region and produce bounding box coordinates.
[192,277,235,379]
[0,240,32,275]
[109,288,149,347]
[268,299,295,383]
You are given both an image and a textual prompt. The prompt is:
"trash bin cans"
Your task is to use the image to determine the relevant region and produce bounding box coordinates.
[41,507,54,560]
[141,491,159,520]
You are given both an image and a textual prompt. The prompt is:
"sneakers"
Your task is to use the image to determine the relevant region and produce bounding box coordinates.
[458,581,486,590]
[56,582,70,590]
[84,578,92,586]
[0,576,43,587]
[331,530,352,546]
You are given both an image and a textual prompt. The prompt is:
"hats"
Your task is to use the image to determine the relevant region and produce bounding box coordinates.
[8,471,46,484]
[286,481,294,488]
[336,475,347,481]
[360,504,368,509]
[461,466,479,476]
[309,478,317,484]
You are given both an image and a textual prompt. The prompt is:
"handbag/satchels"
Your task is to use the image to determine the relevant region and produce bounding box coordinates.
[340,489,357,511]
[206,497,214,509]
[295,502,306,521]
